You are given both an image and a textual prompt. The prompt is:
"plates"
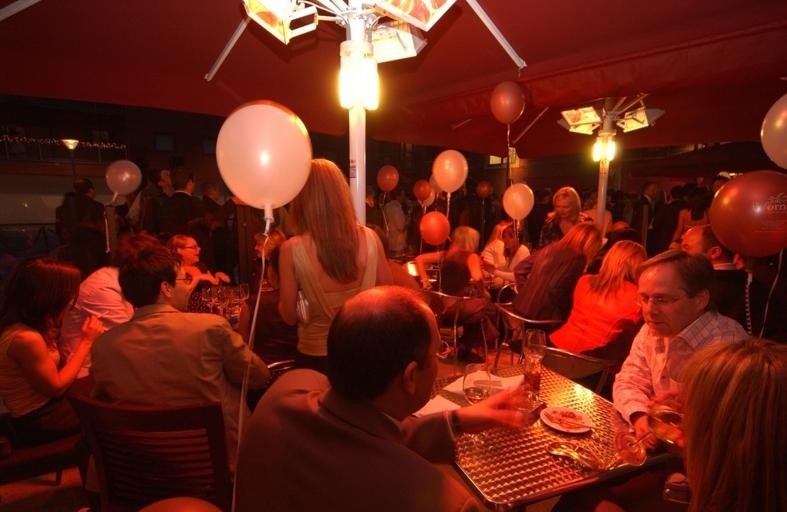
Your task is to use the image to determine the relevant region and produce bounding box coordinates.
[540,407,593,433]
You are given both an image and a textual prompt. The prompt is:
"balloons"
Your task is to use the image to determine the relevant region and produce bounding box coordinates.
[217,102,315,209]
[757,90,787,173]
[487,72,528,128]
[430,150,469,195]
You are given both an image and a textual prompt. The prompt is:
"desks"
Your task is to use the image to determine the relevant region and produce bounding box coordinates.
[419,360,674,512]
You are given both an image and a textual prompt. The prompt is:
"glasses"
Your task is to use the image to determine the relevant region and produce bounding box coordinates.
[432,340,451,359]
[635,293,688,306]
[175,272,192,284]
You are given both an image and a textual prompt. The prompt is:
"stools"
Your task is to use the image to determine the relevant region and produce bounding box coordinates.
[0,436,89,493]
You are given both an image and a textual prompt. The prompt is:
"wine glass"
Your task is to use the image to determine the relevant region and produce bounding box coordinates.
[462,364,490,441]
[512,329,545,409]
[203,283,250,329]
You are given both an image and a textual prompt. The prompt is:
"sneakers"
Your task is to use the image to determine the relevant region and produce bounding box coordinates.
[457,348,485,363]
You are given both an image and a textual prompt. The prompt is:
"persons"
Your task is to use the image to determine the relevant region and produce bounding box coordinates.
[1,157,787,510]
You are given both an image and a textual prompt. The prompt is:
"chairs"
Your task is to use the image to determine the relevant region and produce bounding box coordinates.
[491,302,564,378]
[537,344,618,406]
[424,290,488,362]
[70,392,234,512]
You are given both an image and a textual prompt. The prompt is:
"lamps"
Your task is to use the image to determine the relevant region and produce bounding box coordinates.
[554,93,664,164]
[237,0,459,116]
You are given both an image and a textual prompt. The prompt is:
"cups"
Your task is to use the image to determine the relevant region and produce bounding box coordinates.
[521,360,540,394]
